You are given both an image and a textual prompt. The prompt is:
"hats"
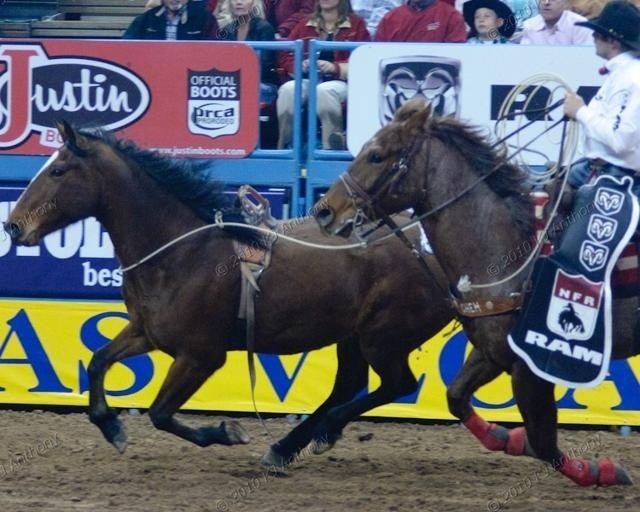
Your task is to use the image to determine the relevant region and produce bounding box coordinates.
[463,0,516,37]
[574,1,640,49]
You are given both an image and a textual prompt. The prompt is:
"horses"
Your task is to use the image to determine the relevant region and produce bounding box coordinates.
[312,93,640,487]
[3,118,458,468]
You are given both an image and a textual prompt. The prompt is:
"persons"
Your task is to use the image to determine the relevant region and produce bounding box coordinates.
[545,0,640,214]
[120,0,640,151]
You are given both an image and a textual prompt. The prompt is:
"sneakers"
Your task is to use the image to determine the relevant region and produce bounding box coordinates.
[327,132,343,150]
